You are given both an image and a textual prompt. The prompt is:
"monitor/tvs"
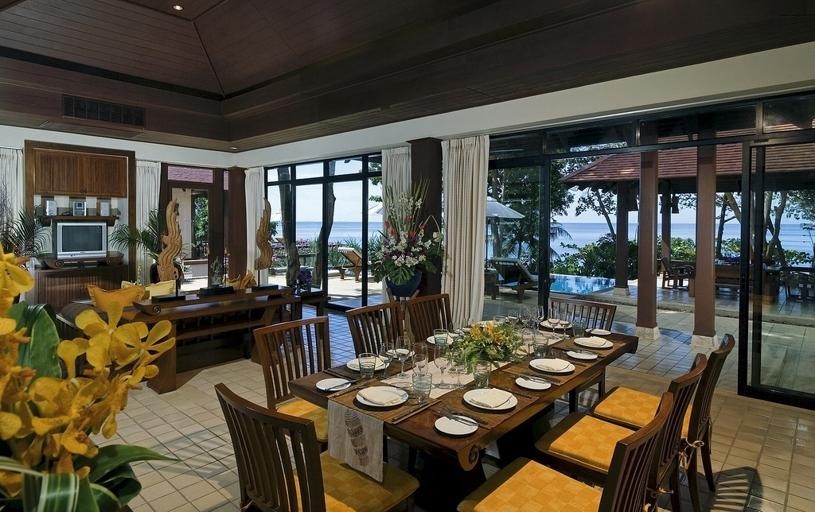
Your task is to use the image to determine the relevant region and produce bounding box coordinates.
[56,221,107,271]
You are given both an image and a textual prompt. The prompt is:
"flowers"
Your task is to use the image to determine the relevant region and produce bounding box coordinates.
[368,178,447,286]
[0,241,182,512]
[449,318,528,375]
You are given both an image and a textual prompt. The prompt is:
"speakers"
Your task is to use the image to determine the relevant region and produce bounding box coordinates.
[45,200,57,216]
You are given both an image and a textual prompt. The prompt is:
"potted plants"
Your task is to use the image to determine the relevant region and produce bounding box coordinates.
[110,211,182,292]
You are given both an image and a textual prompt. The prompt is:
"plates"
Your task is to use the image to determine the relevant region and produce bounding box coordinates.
[387,349,415,359]
[540,320,572,329]
[456,327,471,335]
[356,386,409,407]
[567,350,598,360]
[477,321,503,328]
[463,388,518,411]
[530,358,576,373]
[585,328,611,335]
[427,333,458,345]
[435,415,479,436]
[516,377,551,390]
[347,357,390,372]
[315,377,351,391]
[574,337,613,348]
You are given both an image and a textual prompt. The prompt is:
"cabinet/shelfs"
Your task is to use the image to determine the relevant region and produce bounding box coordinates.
[132,286,301,394]
[38,264,128,314]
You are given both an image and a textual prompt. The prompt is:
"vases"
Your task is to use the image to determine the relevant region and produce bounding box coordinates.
[471,361,491,390]
[385,270,422,297]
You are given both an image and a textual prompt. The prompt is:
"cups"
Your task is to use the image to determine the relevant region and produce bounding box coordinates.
[413,344,429,368]
[560,312,571,337]
[395,336,410,378]
[434,329,447,343]
[473,361,490,388]
[574,320,585,337]
[493,314,506,325]
[358,353,376,379]
[413,354,429,382]
[452,348,466,382]
[534,307,544,328]
[520,307,531,324]
[412,374,431,405]
[530,306,539,328]
[533,337,548,357]
[379,343,393,378]
[509,311,520,330]
[547,310,560,333]
[434,345,450,389]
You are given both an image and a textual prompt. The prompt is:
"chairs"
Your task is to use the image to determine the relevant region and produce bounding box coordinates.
[590,334,736,512]
[534,353,708,512]
[457,392,674,512]
[253,316,331,452]
[405,294,453,343]
[338,247,372,281]
[490,256,556,303]
[345,302,404,358]
[660,256,687,288]
[214,382,420,512]
[550,297,617,414]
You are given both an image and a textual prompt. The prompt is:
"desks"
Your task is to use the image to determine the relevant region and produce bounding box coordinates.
[484,269,499,294]
[288,318,639,493]
[689,263,740,301]
